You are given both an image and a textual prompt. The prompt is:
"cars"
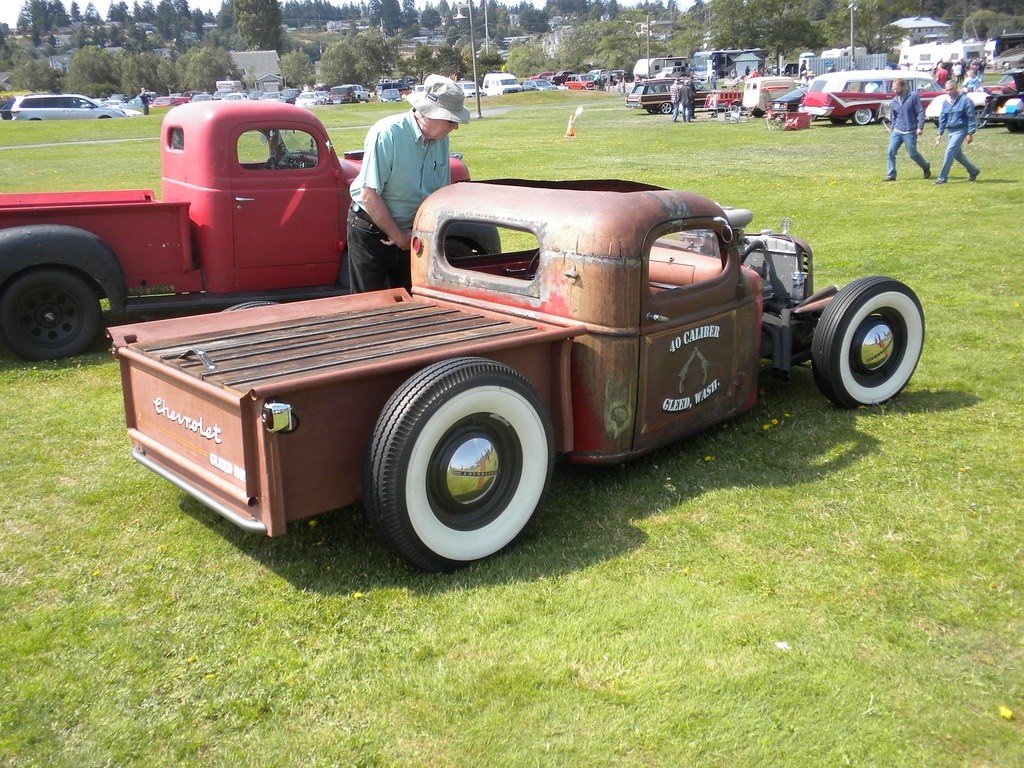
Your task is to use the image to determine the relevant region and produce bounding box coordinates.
[797,69,946,126]
[80,104,145,118]
[106,94,128,103]
[127,81,371,107]
[0,98,15,120]
[925,86,990,129]
[977,68,1024,133]
[103,101,126,107]
[11,94,126,121]
[624,63,807,119]
[376,69,624,103]
[81,99,101,108]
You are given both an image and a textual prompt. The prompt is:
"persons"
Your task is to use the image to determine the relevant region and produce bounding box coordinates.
[670,77,697,124]
[731,66,766,77]
[347,74,470,296]
[800,60,806,80]
[593,75,625,94]
[710,70,716,89]
[881,79,931,181]
[139,87,151,115]
[933,79,981,185]
[935,57,986,93]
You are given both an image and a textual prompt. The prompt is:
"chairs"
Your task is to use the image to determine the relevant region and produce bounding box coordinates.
[741,107,754,121]
[766,117,783,131]
[730,107,740,124]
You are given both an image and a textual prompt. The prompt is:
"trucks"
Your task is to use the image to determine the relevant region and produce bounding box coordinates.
[633,57,694,79]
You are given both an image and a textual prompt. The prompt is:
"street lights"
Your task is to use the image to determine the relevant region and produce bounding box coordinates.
[453,5,484,119]
[848,3,857,70]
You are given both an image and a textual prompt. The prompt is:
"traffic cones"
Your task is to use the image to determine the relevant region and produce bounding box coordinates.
[565,115,575,138]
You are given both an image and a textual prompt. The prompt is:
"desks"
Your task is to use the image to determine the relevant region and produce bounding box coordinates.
[773,111,811,129]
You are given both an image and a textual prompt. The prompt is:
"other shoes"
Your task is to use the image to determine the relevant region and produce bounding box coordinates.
[969,171,980,180]
[924,163,930,179]
[935,180,946,184]
[884,176,896,181]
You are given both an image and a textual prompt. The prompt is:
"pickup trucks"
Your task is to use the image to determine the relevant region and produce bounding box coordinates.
[104,177,926,574]
[0,100,471,360]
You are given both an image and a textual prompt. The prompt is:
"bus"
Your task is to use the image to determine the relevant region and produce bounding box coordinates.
[692,48,768,80]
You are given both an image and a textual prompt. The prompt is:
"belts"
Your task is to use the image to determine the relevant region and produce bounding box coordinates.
[351,202,373,223]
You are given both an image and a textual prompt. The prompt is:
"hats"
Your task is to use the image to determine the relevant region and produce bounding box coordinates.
[405,74,471,124]
